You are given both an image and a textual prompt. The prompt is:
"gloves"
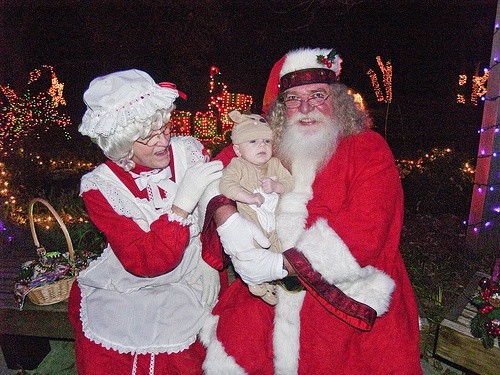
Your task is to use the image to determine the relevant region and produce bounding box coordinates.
[231,249,289,286]
[187,256,222,307]
[172,160,224,214]
[215,211,271,258]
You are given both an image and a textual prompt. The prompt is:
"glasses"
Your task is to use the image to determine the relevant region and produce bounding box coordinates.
[137,121,174,147]
[281,94,332,110]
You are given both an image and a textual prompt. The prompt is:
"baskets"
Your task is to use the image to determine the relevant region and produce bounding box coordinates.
[18,198,100,306]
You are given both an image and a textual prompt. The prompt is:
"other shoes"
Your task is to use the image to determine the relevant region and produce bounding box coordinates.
[248,283,267,297]
[260,285,279,305]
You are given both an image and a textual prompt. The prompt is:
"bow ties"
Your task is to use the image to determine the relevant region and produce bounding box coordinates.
[134,163,177,210]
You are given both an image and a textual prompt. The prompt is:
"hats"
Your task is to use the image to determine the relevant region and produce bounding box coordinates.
[78,68,187,139]
[261,48,343,117]
[228,111,273,143]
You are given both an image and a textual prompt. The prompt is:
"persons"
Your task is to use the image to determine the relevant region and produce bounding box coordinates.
[67,69,223,375]
[198,47,423,375]
[220,110,295,306]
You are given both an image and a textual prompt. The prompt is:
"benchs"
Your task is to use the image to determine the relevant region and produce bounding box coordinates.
[0,254,84,370]
[433,270,500,374]
